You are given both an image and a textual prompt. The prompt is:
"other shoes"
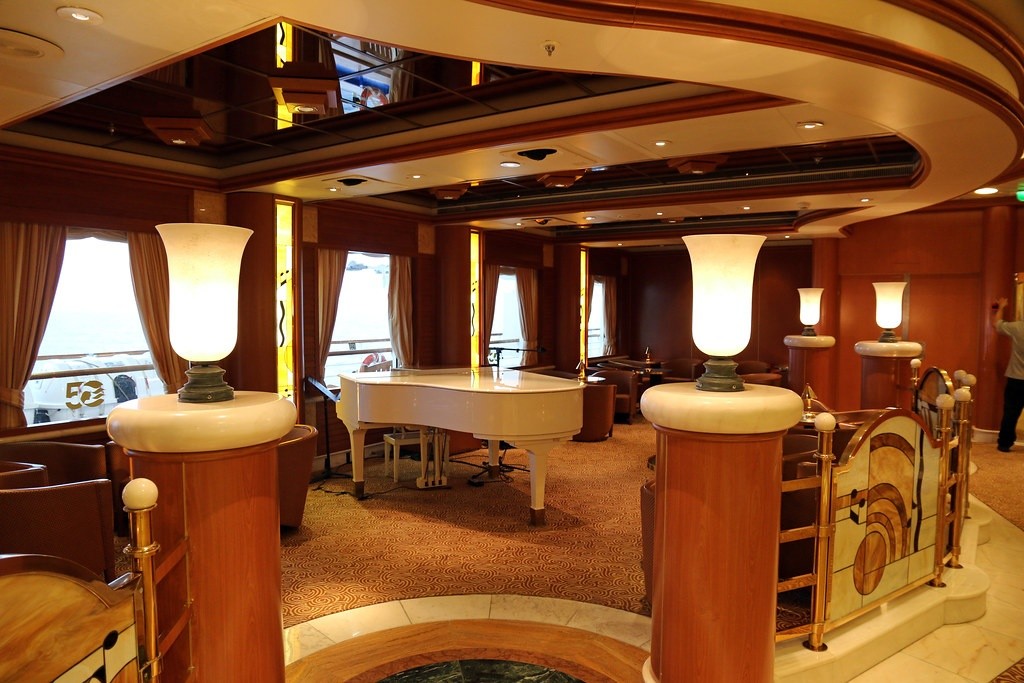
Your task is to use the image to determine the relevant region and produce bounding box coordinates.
[996,439,1014,453]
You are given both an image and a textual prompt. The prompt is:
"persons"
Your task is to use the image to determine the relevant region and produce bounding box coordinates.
[992,297,1024,452]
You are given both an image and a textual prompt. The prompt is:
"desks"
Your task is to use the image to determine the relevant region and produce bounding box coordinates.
[740,373,782,387]
[790,423,861,463]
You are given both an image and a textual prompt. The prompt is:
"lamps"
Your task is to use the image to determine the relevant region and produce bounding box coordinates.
[797,288,825,336]
[155,222,256,404]
[681,234,768,392]
[873,282,908,344]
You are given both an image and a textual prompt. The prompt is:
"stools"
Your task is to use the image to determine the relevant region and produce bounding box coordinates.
[0,424,320,583]
[383,430,450,484]
[640,409,891,605]
[537,358,780,443]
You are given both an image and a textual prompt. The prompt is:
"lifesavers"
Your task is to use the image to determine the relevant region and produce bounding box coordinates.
[360,352,389,372]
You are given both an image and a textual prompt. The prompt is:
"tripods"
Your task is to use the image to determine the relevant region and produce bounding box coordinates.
[306,376,353,485]
[471,347,546,479]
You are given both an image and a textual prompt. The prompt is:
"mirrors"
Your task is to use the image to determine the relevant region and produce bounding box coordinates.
[0,16,677,170]
[373,134,922,209]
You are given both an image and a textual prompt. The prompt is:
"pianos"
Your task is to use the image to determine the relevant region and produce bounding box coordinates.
[335,365,587,526]
